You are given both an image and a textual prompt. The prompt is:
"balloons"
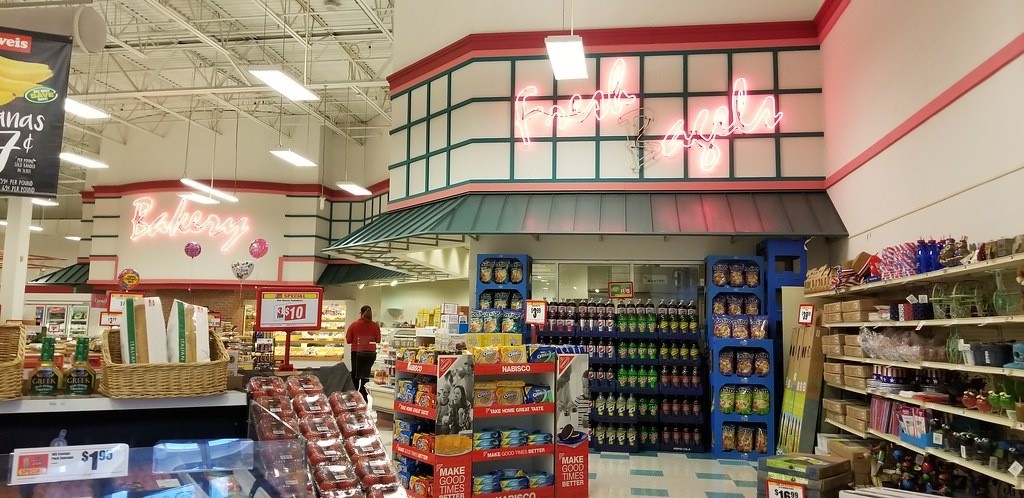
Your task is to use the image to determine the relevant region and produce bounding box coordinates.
[184,241,202,258]
[231,260,254,282]
[117,268,140,291]
[250,238,269,259]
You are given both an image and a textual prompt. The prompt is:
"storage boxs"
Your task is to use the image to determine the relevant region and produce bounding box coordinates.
[755,299,886,498]
[467,332,585,494]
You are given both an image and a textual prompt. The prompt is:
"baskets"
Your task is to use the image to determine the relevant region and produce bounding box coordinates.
[97,329,230,399]
[0,323,26,401]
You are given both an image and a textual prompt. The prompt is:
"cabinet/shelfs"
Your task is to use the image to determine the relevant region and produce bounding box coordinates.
[536,235,807,491]
[805,252,1024,490]
[477,254,532,332]
[391,344,589,498]
[243,241,468,498]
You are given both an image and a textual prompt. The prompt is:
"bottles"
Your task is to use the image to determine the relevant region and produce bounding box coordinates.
[579,298,616,332]
[640,425,702,447]
[587,337,615,359]
[658,299,698,334]
[62,337,96,394]
[618,365,637,388]
[617,299,657,333]
[638,365,658,388]
[595,392,657,417]
[660,366,680,388]
[588,364,615,387]
[681,366,701,389]
[28,337,62,396]
[617,339,657,360]
[537,297,577,332]
[660,341,698,360]
[541,336,586,354]
[596,423,636,446]
[661,397,701,418]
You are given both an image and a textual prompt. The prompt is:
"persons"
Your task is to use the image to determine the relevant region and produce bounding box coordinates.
[346,304,381,404]
[437,383,470,435]
